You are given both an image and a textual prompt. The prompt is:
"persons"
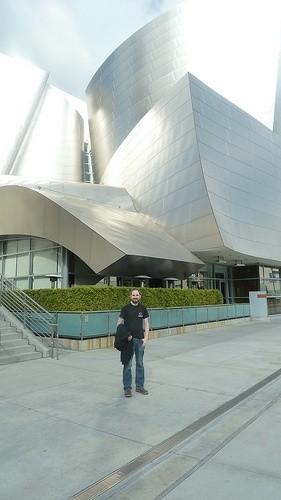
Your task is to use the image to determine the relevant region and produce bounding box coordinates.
[117,289,149,397]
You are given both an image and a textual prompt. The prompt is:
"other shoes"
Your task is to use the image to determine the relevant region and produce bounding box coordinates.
[136,386,148,395]
[124,388,132,397]
